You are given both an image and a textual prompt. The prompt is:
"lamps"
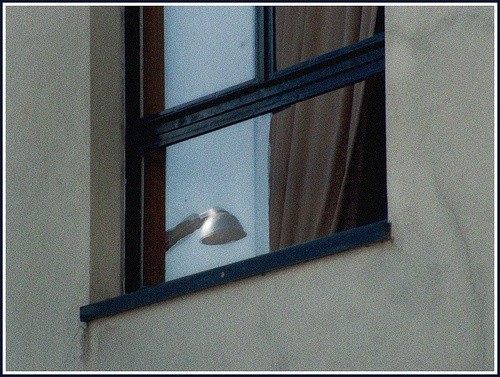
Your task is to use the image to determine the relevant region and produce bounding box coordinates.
[162,203,247,253]
